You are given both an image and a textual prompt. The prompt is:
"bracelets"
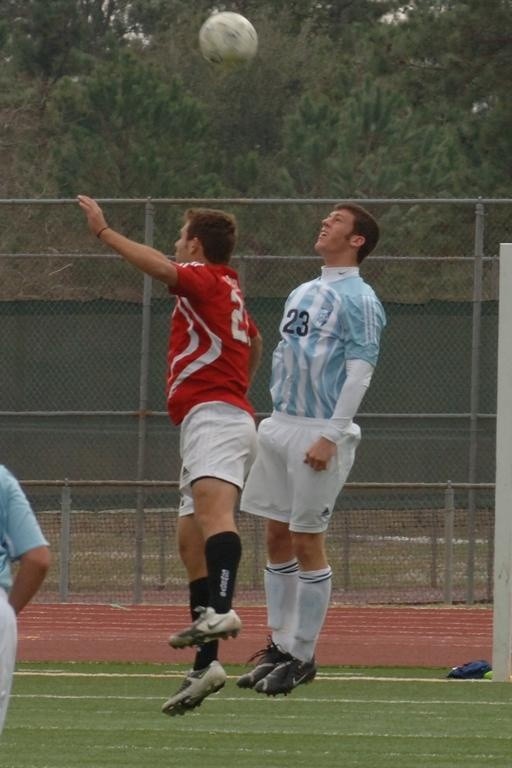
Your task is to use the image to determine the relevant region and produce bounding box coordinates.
[95,224,111,240]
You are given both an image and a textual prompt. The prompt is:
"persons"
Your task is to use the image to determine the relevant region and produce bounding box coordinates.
[233,201,388,697]
[74,186,265,718]
[0,456,54,743]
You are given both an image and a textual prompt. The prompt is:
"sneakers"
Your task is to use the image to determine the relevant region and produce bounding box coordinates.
[234,642,296,692]
[251,658,326,702]
[164,602,243,650]
[159,658,229,717]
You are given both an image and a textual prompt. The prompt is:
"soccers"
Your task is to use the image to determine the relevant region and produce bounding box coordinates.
[199,11,257,69]
